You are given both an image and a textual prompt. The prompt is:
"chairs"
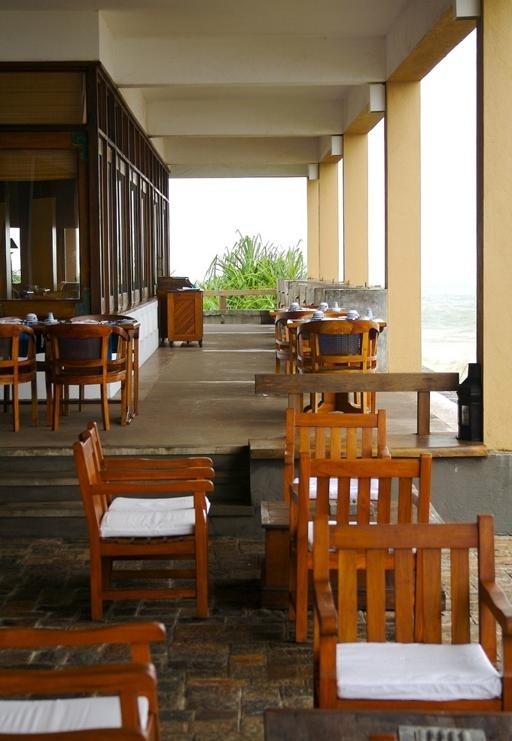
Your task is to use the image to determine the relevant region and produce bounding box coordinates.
[0,315,141,428]
[1,622,166,740]
[73,421,215,620]
[313,510,510,713]
[283,410,388,505]
[272,303,387,417]
[295,452,433,639]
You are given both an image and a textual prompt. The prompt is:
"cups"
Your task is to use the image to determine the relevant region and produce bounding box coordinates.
[27,313,38,321]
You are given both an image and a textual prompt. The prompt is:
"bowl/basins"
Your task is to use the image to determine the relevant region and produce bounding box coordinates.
[288,302,358,319]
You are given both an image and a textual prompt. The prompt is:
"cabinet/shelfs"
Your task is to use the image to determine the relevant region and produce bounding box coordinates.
[168,289,203,342]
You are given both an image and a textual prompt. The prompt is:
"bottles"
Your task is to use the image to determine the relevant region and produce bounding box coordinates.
[48,312,54,321]
